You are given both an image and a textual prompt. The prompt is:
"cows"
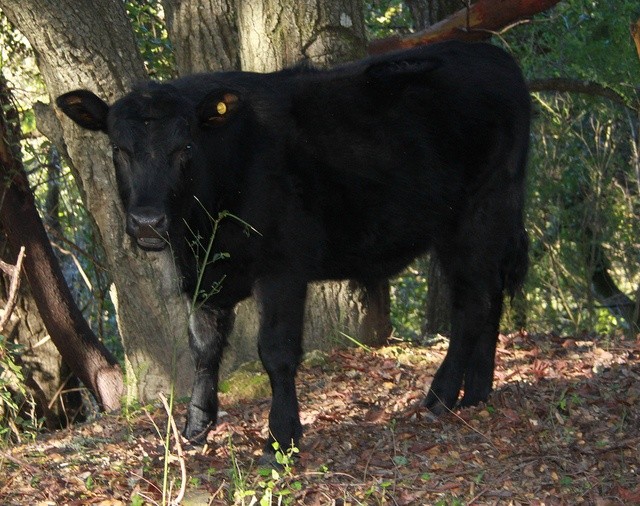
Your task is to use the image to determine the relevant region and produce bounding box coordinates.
[55,31,531,452]
